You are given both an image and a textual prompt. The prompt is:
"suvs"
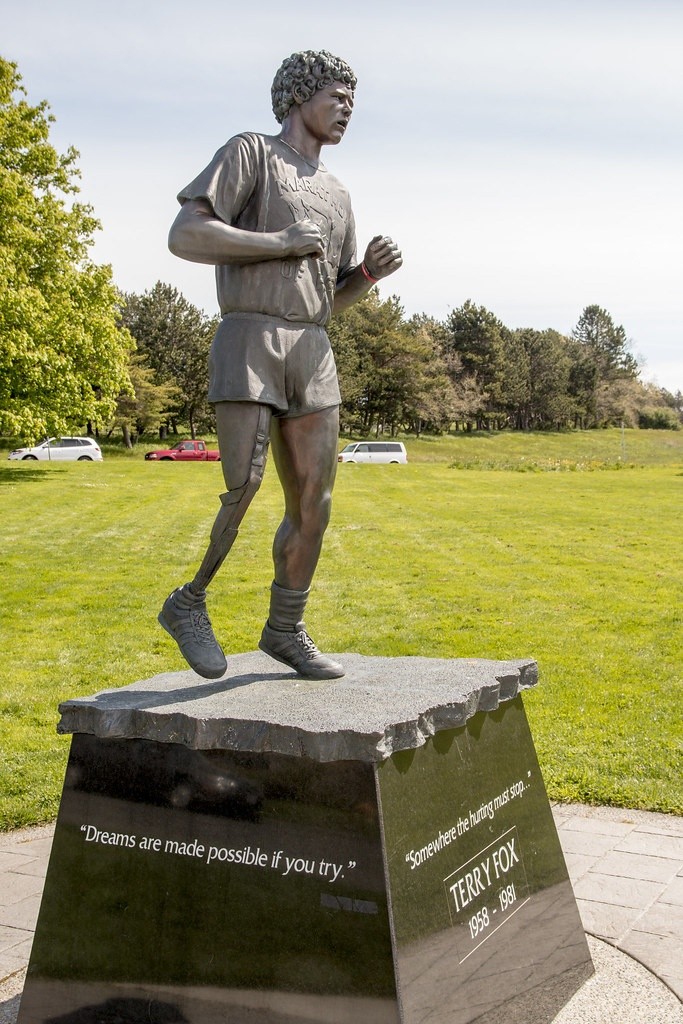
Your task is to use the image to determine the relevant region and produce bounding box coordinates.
[8,436,103,462]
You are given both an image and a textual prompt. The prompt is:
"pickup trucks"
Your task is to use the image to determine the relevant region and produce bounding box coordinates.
[145,440,221,461]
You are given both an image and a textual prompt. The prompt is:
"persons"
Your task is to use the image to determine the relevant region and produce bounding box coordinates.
[155,48,404,682]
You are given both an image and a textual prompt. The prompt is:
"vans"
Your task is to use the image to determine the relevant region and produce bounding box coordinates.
[338,442,408,464]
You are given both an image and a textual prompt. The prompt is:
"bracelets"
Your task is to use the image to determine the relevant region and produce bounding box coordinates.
[360,261,381,285]
